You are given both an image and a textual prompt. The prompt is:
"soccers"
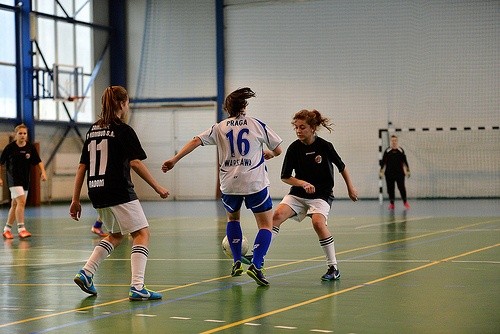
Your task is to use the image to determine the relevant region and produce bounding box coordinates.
[222,233,249,260]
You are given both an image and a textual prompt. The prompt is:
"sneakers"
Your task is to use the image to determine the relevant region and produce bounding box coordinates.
[246,263,271,288]
[73,269,97,295]
[320,265,341,281]
[3,230,14,239]
[240,254,264,268]
[18,230,32,239]
[91,226,108,237]
[128,284,162,301]
[230,260,244,277]
[388,204,395,210]
[403,201,410,208]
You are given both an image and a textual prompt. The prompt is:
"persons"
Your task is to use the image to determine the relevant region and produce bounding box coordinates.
[241,109,357,281]
[0,124,48,238]
[70,86,170,301]
[162,87,283,286]
[380,135,410,208]
